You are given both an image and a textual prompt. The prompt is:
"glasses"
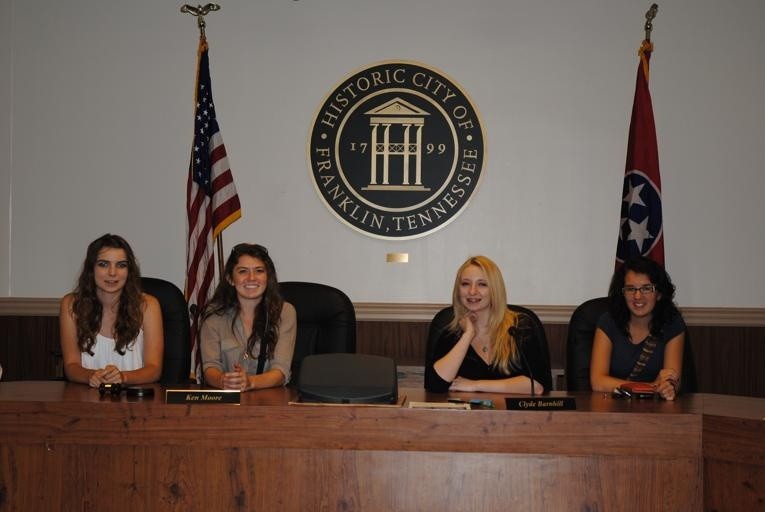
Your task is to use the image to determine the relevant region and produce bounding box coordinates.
[622,285,655,294]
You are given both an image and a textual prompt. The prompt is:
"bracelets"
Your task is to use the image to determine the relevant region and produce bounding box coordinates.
[666,374,681,393]
[218,372,225,388]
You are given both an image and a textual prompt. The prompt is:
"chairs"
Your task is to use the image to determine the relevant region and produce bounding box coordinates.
[277,281,356,380]
[136,277,192,384]
[424,305,552,392]
[565,297,698,393]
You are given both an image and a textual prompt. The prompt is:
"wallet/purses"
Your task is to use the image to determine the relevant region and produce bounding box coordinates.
[620,383,654,393]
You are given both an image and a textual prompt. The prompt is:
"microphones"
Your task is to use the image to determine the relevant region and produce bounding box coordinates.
[189,303,205,390]
[508,326,535,396]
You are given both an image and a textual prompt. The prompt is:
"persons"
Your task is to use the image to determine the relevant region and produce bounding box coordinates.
[196,240,298,391]
[588,256,690,403]
[58,234,165,389]
[423,254,553,397]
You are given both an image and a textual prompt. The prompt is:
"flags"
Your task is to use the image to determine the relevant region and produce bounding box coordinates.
[178,2,242,380]
[612,1,669,279]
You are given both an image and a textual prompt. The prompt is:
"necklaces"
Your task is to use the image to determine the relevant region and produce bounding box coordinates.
[475,334,494,353]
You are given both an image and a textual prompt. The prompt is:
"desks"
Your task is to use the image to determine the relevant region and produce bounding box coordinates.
[0,381,763,511]
